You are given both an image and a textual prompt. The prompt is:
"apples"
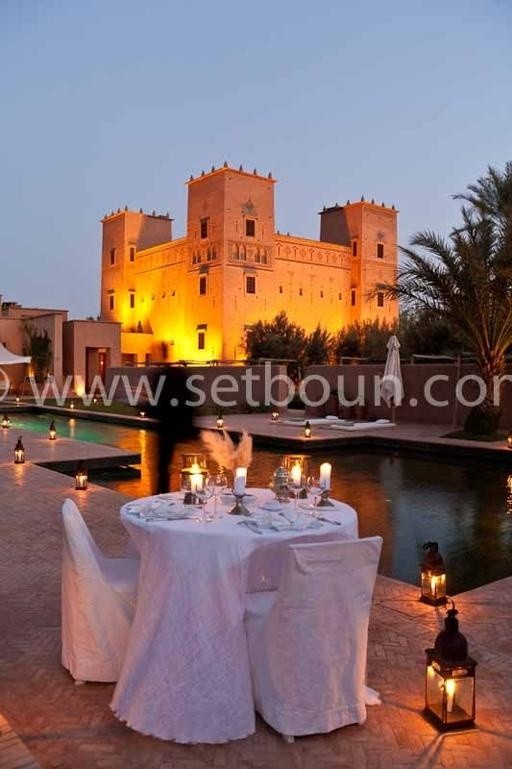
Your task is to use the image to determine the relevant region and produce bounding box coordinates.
[290,462,331,489]
[190,466,247,495]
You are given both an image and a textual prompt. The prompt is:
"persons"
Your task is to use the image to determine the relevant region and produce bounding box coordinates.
[142,342,201,493]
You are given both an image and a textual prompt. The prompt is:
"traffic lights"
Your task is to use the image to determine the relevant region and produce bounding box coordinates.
[326,416,337,420]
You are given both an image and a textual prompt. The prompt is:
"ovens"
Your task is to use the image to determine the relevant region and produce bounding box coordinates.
[284,418,397,431]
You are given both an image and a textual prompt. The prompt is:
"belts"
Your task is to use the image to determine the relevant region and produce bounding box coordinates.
[288,474,326,518]
[194,475,228,526]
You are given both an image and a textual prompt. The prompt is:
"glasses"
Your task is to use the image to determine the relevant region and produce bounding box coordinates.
[415,539,479,733]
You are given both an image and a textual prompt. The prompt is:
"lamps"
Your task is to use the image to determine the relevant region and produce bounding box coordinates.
[60,497,140,685]
[245,536,385,745]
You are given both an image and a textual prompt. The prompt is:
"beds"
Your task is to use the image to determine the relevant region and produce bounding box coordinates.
[0,342,33,365]
[380,332,405,424]
